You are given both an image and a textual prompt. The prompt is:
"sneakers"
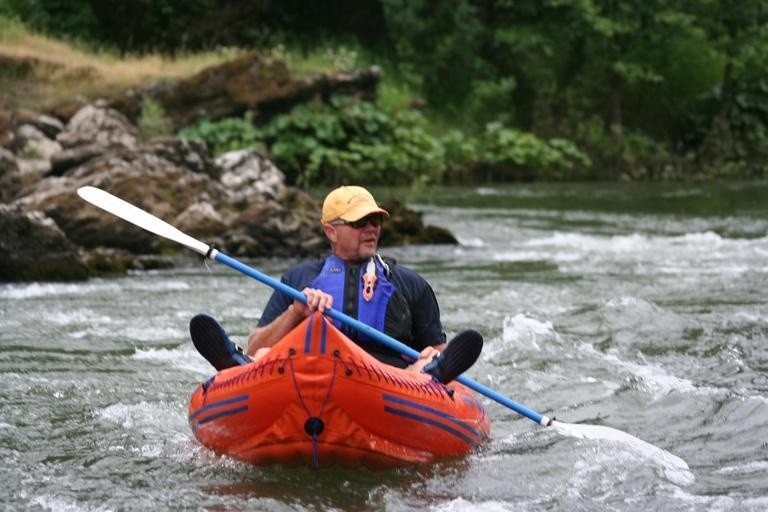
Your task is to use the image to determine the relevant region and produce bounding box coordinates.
[187,313,252,371]
[419,327,484,381]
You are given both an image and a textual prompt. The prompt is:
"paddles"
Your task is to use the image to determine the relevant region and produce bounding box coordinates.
[77,185,689,469]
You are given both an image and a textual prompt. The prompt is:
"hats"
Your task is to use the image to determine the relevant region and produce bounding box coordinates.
[319,184,391,227]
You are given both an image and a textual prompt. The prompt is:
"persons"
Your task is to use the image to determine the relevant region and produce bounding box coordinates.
[187,183,482,391]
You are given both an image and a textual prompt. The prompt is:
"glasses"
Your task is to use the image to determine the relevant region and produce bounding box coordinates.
[330,213,384,228]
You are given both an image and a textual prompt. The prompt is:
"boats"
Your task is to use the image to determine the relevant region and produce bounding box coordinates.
[187,309,491,468]
